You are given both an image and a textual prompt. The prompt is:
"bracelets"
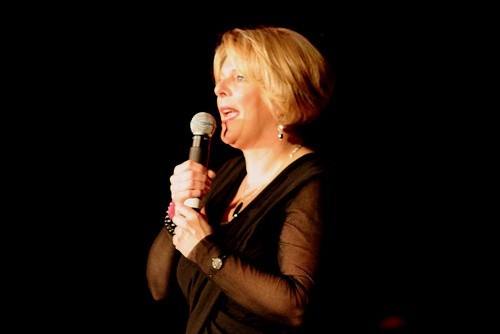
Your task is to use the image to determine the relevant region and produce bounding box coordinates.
[163,214,177,237]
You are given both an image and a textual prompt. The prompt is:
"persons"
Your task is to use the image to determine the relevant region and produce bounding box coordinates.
[147,27,336,334]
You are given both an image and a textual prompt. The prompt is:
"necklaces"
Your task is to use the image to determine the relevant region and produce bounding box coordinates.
[232,144,302,217]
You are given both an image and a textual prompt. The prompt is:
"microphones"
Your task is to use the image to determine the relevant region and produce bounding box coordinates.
[184,112,217,214]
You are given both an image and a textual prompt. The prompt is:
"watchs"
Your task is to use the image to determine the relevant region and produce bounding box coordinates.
[206,254,227,279]
